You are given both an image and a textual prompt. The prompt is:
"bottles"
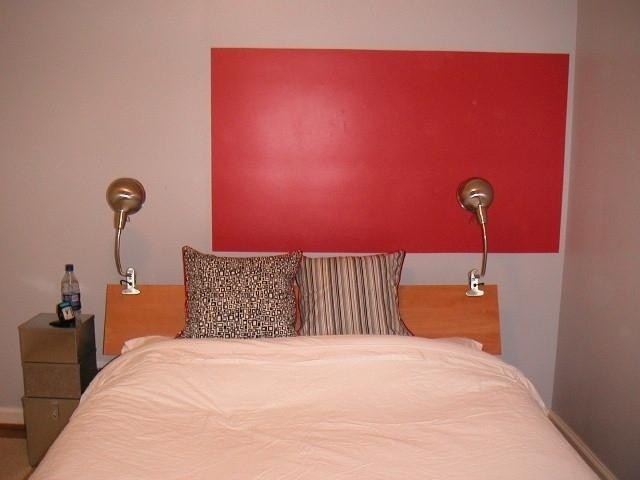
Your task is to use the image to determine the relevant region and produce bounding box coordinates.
[59,264,82,324]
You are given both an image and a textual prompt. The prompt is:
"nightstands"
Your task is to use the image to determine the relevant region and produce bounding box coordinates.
[18,313,98,468]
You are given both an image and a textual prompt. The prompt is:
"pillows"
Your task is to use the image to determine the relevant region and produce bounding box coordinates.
[295,252,417,332]
[176,244,305,342]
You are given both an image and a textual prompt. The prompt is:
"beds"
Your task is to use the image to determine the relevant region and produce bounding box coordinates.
[24,282,602,480]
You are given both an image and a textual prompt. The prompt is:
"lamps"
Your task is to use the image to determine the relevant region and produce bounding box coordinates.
[459,178,496,298]
[106,179,147,297]
